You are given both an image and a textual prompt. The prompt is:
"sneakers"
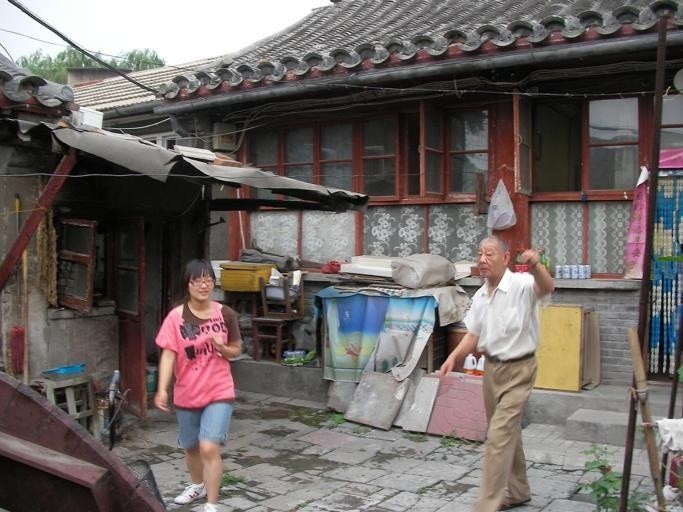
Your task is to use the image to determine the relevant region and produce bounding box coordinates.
[174,481,206,505]
[203,503,217,512]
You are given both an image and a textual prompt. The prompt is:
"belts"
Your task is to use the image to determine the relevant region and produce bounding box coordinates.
[485,351,537,363]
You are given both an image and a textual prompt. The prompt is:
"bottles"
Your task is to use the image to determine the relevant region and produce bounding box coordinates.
[514,248,546,273]
[284,351,304,358]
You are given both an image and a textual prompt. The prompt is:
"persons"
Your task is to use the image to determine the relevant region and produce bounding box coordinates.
[439,235,555,511]
[154,259,242,512]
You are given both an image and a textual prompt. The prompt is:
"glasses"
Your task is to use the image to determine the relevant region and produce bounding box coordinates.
[189,280,215,286]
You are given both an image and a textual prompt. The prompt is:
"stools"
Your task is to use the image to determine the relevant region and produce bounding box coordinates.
[230,293,266,361]
[252,316,293,363]
[33,375,96,438]
[259,274,305,319]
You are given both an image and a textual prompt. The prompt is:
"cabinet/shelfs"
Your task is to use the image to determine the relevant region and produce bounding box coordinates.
[532,305,600,392]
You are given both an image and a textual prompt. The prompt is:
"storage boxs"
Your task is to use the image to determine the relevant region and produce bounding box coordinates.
[219,261,276,293]
